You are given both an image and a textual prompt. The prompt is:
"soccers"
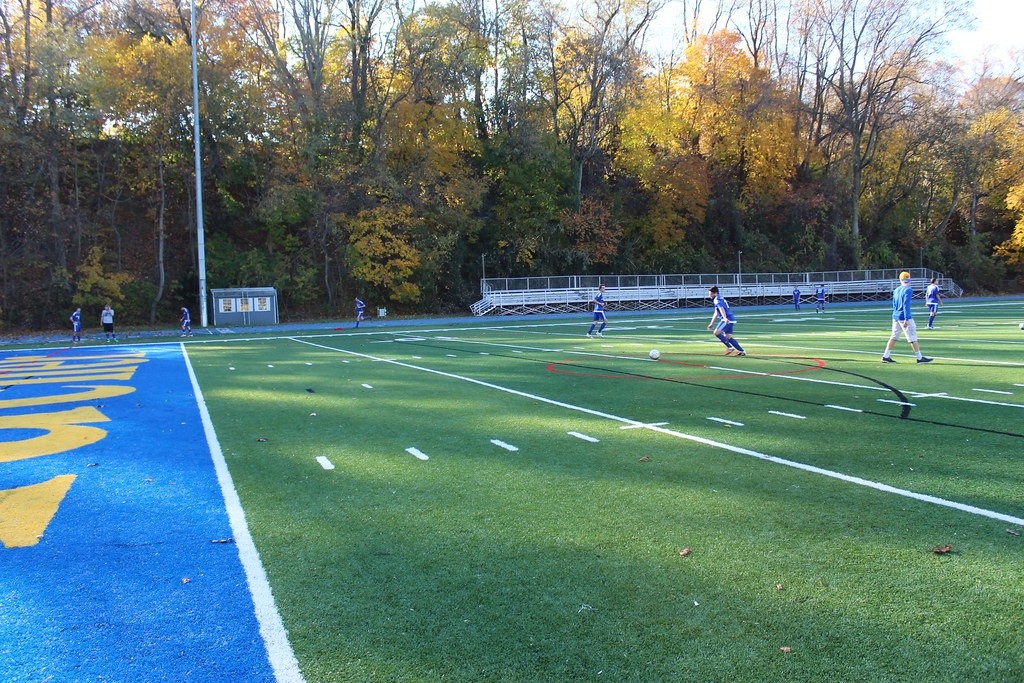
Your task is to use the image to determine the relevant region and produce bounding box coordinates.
[648,349,661,360]
[1019,322,1024,330]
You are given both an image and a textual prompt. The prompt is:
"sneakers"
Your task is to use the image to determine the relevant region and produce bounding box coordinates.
[733,351,747,356]
[882,356,896,363]
[917,357,933,364]
[724,346,735,355]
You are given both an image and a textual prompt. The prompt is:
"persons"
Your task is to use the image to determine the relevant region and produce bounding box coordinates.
[815,284,826,314]
[100,304,119,343]
[178,305,194,337]
[586,284,608,339]
[793,287,801,311]
[925,278,943,329]
[354,297,371,328]
[70,306,82,344]
[707,285,746,356]
[880,271,935,364]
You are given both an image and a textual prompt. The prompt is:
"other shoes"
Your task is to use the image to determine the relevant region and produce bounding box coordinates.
[187,334,193,338]
[822,310,824,314]
[114,338,118,342]
[181,334,186,338]
[816,308,819,313]
[926,323,934,330]
[596,331,604,338]
[587,333,593,339]
[107,339,110,343]
